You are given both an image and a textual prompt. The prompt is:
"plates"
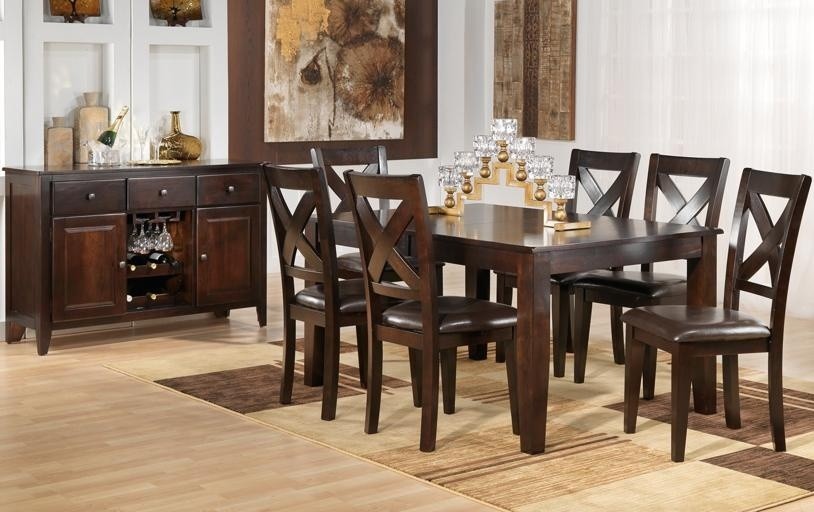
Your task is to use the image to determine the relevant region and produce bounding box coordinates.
[49,0,102,17]
[150,0,205,19]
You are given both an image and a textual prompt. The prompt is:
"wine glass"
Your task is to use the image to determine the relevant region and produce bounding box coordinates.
[133,122,165,164]
[129,216,175,254]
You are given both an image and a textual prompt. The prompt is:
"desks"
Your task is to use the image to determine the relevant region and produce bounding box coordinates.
[301,199,725,454]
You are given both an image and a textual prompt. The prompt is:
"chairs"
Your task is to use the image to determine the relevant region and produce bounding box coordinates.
[341,169,522,451]
[492,148,643,379]
[257,158,426,422]
[309,144,448,386]
[618,167,812,462]
[569,151,731,384]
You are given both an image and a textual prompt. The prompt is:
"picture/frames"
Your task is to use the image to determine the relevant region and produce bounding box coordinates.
[226,0,440,164]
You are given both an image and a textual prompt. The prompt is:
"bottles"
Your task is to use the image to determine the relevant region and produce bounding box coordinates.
[128,251,172,264]
[98,104,129,150]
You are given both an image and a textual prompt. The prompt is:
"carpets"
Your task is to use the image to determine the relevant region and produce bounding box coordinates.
[95,329,813,510]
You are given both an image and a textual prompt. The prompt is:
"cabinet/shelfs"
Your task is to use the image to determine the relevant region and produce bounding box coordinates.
[23,0,227,170]
[0,161,271,357]
